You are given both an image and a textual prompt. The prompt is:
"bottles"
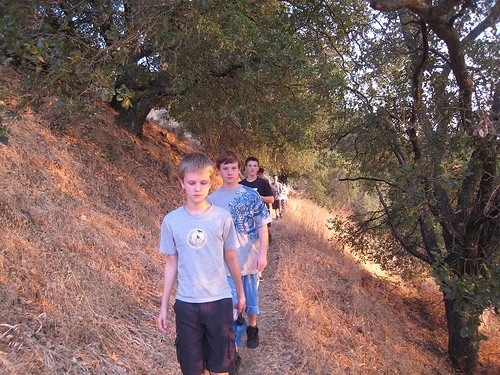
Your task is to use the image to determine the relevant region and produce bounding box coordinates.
[234,312,247,345]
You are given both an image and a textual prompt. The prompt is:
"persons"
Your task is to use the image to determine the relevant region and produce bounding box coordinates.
[209,151,271,348]
[235,157,292,246]
[155,152,248,375]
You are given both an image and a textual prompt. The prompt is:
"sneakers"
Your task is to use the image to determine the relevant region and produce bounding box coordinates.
[275,213,283,222]
[237,312,245,324]
[246,326,259,349]
